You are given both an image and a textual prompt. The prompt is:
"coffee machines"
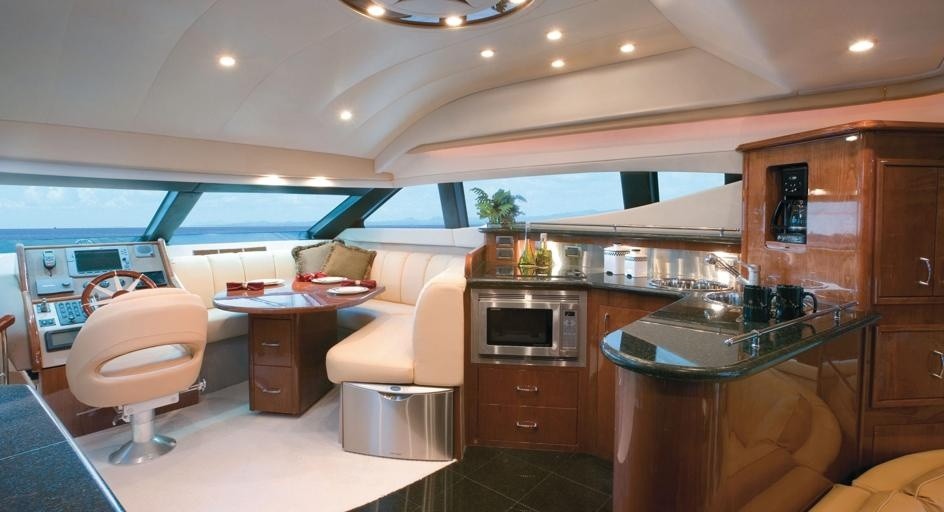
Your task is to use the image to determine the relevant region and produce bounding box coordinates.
[770,168,807,243]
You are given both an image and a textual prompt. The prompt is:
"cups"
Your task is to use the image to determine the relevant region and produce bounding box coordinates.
[743,285,783,322]
[776,285,818,320]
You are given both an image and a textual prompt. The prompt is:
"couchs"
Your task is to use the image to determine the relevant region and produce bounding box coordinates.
[176,237,464,460]
[809,448,944,512]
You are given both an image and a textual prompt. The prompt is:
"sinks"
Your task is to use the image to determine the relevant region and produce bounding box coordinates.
[705,288,742,311]
[649,274,732,293]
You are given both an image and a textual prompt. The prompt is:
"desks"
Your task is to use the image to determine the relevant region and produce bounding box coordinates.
[212,276,385,417]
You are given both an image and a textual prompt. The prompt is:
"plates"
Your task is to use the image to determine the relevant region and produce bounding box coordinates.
[245,279,284,286]
[326,286,370,295]
[311,276,348,284]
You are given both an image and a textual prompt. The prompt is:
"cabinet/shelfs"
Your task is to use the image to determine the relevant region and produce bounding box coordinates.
[588,288,674,455]
[735,119,944,468]
[465,363,594,454]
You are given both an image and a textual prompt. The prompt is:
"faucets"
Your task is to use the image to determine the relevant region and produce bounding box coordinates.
[703,252,760,289]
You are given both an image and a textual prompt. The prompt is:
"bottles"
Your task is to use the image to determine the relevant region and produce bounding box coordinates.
[535,233,552,276]
[518,222,536,276]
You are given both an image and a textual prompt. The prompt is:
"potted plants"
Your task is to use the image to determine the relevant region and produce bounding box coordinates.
[471,186,528,228]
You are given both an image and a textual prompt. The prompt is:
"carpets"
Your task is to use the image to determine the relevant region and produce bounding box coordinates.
[347,445,613,512]
[74,379,458,512]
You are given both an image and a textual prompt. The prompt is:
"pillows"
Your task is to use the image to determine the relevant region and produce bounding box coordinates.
[291,240,347,286]
[320,242,376,283]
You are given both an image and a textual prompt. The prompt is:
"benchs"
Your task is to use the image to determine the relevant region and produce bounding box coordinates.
[0,384,128,511]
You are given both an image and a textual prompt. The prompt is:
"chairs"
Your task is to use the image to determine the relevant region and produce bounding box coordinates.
[65,288,208,466]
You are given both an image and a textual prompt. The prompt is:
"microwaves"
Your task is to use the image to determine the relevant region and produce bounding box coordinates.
[470,288,589,368]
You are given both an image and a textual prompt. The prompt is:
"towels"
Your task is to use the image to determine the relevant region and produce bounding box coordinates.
[227,282,265,290]
[341,279,376,287]
[296,271,327,281]
